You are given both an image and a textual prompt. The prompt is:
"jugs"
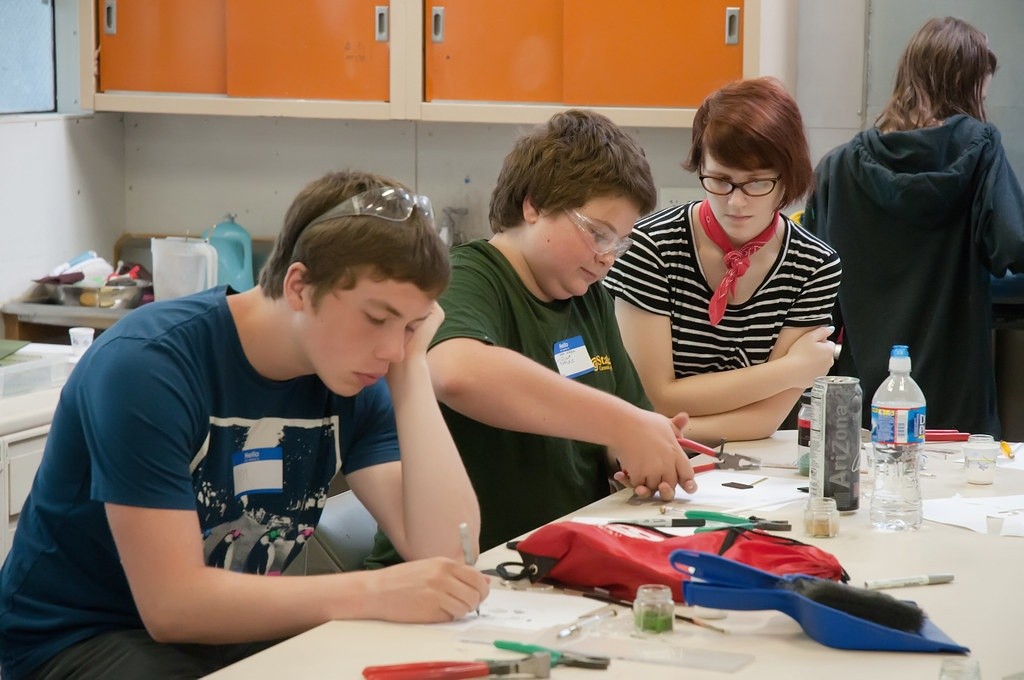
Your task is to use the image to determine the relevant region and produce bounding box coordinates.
[151,236,219,302]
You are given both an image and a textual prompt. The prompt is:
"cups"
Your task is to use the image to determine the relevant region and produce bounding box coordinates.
[962,442,1001,485]
[69,327,95,358]
[864,442,874,479]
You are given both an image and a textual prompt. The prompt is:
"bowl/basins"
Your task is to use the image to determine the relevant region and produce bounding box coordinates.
[31,278,153,309]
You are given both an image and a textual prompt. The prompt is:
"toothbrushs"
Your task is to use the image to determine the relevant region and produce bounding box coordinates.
[106,260,124,284]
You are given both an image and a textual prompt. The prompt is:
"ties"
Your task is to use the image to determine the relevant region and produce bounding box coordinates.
[693,200,781,333]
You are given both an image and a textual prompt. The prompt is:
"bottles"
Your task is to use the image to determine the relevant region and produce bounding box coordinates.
[796,391,812,478]
[802,497,840,539]
[632,583,674,634]
[967,434,995,443]
[868,345,926,533]
[200,213,255,293]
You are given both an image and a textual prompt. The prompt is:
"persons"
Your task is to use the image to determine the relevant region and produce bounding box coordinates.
[426,109,698,556]
[0,169,491,680]
[601,74,842,454]
[801,15,1024,442]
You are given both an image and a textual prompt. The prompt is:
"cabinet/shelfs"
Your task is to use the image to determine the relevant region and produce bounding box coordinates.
[79,2,801,126]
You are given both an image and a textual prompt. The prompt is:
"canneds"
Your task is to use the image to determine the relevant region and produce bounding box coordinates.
[808,377,862,514]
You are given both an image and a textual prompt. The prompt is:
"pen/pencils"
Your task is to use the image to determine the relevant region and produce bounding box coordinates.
[555,610,616,638]
[460,524,483,613]
[868,572,957,592]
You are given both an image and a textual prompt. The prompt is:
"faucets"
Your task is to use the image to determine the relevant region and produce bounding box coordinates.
[437,207,468,245]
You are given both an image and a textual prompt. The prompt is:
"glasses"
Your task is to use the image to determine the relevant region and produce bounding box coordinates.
[560,204,634,260]
[288,186,436,267]
[695,158,784,198]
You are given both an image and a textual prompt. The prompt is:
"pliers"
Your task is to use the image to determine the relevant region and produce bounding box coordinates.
[623,437,760,476]
[362,650,551,680]
[925,428,970,442]
[475,640,610,671]
[685,510,792,534]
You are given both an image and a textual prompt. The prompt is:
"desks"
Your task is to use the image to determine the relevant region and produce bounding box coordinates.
[0,342,98,565]
[200,427,1024,680]
